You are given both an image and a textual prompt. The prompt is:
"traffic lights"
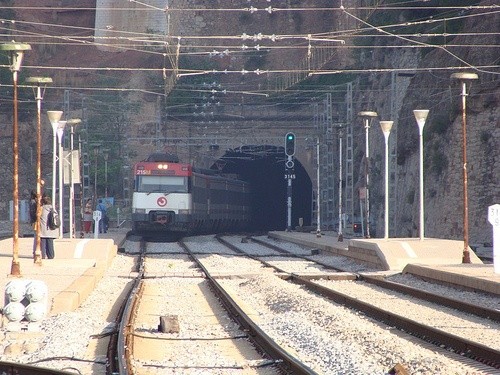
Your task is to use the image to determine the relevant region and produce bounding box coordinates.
[285,132,296,156]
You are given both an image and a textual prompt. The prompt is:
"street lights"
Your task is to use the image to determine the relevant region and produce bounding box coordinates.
[331,120,350,244]
[413,109,431,238]
[46,110,64,208]
[66,118,82,239]
[25,75,53,266]
[0,39,32,279]
[90,143,101,212]
[450,72,479,265]
[100,147,112,198]
[56,120,68,239]
[378,120,395,237]
[357,111,378,238]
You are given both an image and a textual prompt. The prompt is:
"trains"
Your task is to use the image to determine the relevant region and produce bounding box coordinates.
[130,160,255,235]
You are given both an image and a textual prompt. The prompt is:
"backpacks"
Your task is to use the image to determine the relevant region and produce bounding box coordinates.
[40,207,60,230]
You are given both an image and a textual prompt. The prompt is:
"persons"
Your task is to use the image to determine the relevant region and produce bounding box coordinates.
[28,188,59,259]
[96,200,107,233]
[83,199,92,234]
